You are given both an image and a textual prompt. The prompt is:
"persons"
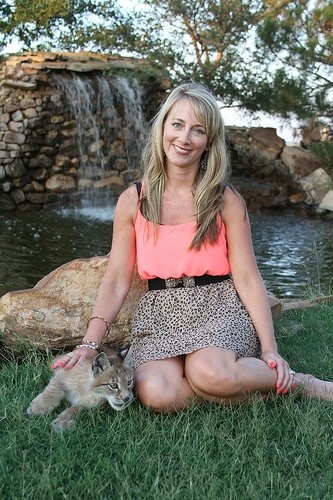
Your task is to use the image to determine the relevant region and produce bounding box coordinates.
[51,84,333,414]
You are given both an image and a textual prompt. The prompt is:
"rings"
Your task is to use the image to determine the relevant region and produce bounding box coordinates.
[288,370,295,375]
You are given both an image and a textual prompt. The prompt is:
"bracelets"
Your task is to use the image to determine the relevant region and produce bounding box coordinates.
[85,316,110,339]
[75,340,100,352]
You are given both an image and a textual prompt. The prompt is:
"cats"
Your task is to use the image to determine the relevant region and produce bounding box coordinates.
[26,345,135,434]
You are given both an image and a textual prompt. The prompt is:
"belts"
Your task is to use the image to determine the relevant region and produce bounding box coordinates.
[148,275,232,290]
